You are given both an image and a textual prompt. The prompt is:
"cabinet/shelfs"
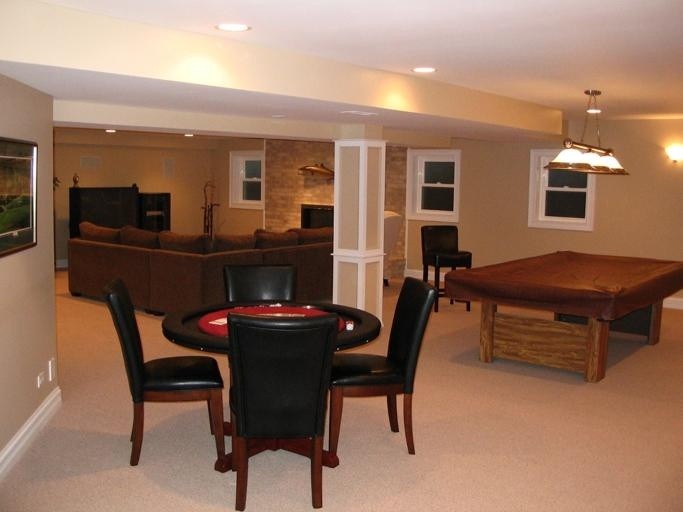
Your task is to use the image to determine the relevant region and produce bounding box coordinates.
[67,184,139,240]
[139,190,171,232]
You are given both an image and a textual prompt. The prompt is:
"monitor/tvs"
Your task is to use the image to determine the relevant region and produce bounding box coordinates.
[301,204,333,229]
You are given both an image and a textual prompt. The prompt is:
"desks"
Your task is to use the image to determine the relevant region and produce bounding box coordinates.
[441,249,683,383]
[161,298,382,468]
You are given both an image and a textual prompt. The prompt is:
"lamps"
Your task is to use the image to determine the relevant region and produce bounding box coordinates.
[541,90,630,173]
[664,142,683,163]
[296,163,333,185]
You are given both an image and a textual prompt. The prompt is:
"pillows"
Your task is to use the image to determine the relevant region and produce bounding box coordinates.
[158,230,216,253]
[254,229,299,248]
[289,226,333,245]
[120,224,159,248]
[213,233,256,251]
[77,221,121,243]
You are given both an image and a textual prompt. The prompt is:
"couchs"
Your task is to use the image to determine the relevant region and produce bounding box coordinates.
[65,237,333,317]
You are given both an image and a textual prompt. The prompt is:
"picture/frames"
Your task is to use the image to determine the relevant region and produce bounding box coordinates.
[0,136,39,258]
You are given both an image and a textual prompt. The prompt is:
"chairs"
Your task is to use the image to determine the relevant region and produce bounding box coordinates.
[419,224,472,315]
[224,313,340,510]
[382,210,403,287]
[102,278,227,468]
[327,276,435,460]
[222,263,297,303]
